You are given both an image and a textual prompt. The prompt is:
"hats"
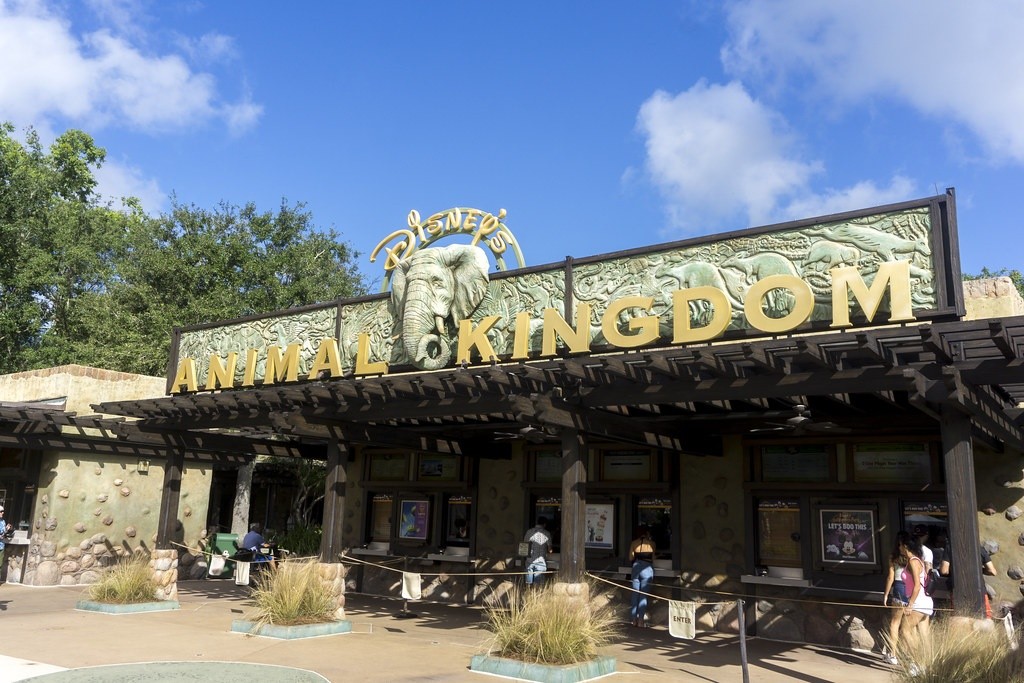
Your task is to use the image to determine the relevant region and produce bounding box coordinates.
[912,524,928,537]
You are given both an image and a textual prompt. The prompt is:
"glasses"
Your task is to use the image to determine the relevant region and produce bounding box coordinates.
[0,510,5,513]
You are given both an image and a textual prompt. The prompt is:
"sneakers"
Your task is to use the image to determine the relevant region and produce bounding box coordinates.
[883,654,897,665]
[906,663,923,678]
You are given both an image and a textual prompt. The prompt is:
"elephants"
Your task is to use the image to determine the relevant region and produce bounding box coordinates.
[390,243,490,371]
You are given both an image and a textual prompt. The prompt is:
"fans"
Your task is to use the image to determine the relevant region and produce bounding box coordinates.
[748,404,853,435]
[493,423,561,443]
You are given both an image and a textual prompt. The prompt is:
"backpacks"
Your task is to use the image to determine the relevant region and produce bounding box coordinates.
[921,569,939,596]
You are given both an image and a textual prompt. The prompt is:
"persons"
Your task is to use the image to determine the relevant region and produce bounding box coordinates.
[0,506,11,580]
[524,516,553,591]
[883,529,997,678]
[628,522,655,628]
[240,523,277,580]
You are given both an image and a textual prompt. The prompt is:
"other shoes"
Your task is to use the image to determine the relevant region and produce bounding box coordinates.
[632,618,650,628]
